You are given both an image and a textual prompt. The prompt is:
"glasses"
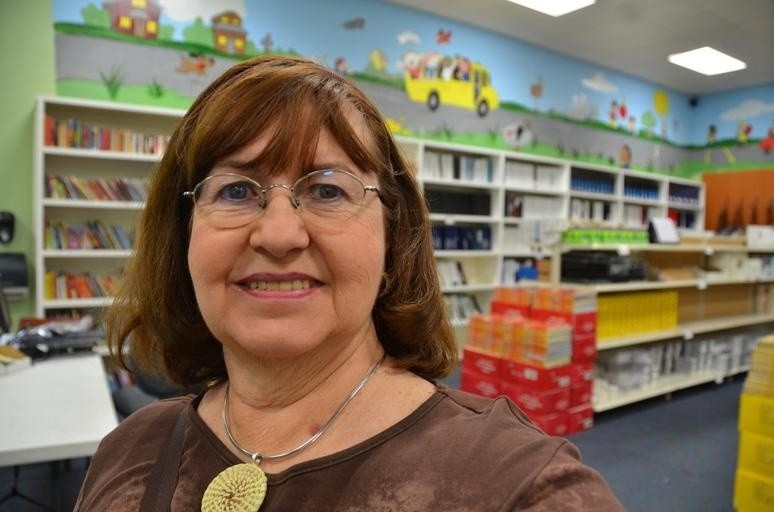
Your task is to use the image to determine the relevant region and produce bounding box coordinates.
[183,167,382,231]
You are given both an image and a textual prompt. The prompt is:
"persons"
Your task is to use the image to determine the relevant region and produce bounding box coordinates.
[73,56,624,512]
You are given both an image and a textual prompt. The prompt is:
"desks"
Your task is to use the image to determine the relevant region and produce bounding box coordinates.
[0,346,122,511]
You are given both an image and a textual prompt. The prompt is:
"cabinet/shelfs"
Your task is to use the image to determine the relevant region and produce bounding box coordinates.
[32,94,707,363]
[515,243,774,414]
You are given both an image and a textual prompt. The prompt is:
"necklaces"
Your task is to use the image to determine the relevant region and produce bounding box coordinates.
[201,351,390,512]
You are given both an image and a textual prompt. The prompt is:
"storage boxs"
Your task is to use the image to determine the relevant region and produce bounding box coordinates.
[459,300,597,437]
